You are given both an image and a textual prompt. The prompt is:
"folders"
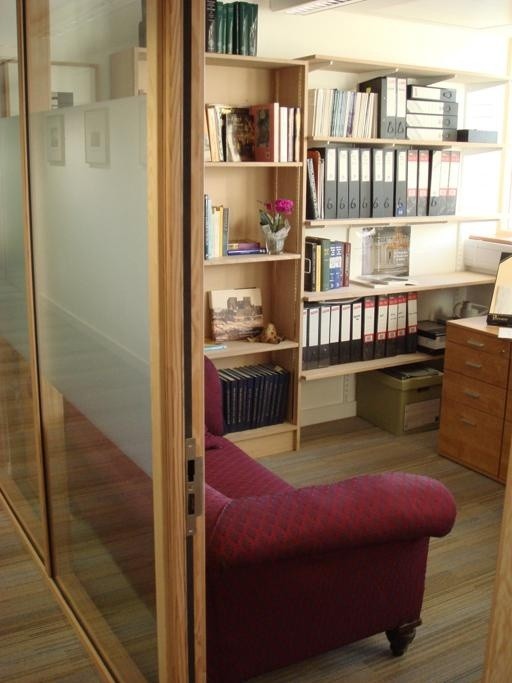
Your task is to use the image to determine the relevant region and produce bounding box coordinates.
[357,75,499,143]
[325,147,460,217]
[301,293,419,370]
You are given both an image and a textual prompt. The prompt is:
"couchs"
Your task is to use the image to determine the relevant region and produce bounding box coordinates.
[64,355,457,682]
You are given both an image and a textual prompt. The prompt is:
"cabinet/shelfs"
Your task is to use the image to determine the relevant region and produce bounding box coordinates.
[291,53,512,426]
[437,315,511,487]
[203,53,309,458]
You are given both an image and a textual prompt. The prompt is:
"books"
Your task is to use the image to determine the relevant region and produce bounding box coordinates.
[204,1,260,58]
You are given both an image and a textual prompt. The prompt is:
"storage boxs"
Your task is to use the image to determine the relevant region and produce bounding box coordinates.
[355,367,443,437]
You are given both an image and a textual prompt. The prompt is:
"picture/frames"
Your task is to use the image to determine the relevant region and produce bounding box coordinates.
[1,58,100,119]
